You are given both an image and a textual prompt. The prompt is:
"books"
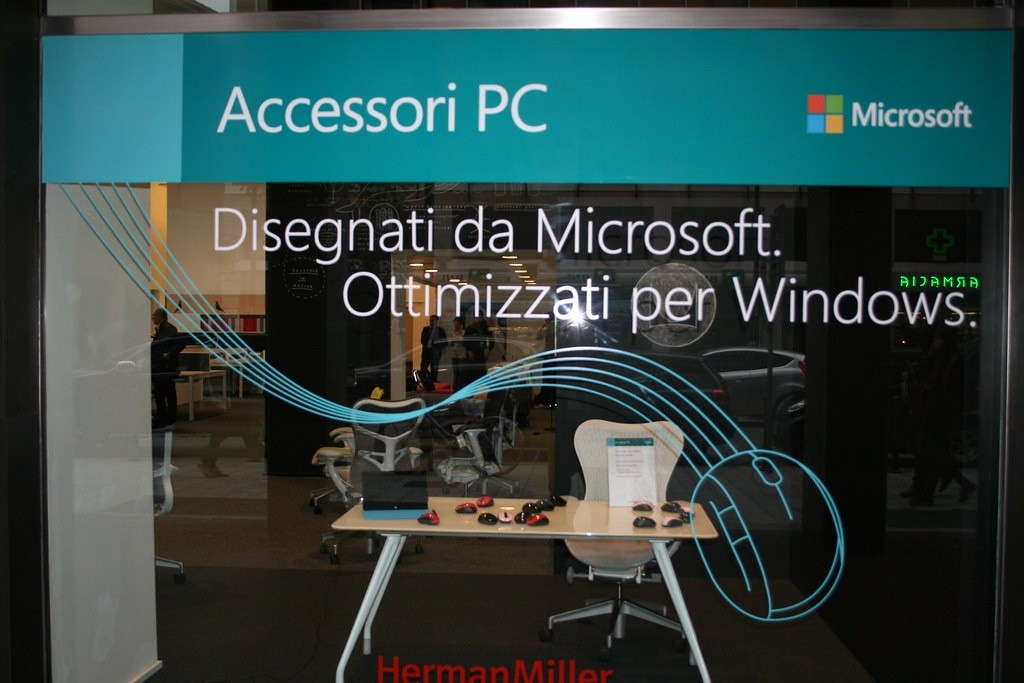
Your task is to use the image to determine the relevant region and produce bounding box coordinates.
[201,316,266,332]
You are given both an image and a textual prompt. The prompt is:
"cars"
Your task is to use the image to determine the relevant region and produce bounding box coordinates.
[587,355,737,467]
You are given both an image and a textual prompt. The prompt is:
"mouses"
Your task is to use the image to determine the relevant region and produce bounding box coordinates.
[417,513,440,524]
[478,513,497,525]
[455,502,478,513]
[499,495,567,524]
[631,500,695,528]
[476,495,494,506]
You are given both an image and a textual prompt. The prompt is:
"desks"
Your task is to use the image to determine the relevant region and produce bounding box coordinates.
[208,359,262,399]
[180,370,228,422]
[331,495,719,683]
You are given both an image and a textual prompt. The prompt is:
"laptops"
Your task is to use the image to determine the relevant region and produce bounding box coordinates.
[362,470,429,520]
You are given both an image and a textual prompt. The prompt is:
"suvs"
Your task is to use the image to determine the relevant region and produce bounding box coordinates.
[344,334,542,433]
[52,331,267,428]
[644,345,806,427]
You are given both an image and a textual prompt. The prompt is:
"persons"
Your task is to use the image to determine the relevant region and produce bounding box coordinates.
[420,315,449,383]
[534,311,555,397]
[151,308,186,429]
[449,309,495,399]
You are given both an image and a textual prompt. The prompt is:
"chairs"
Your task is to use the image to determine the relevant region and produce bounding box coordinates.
[151,425,186,584]
[537,418,698,670]
[309,387,385,514]
[436,396,521,497]
[319,397,424,565]
